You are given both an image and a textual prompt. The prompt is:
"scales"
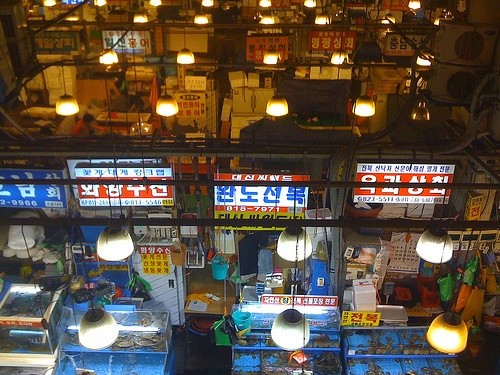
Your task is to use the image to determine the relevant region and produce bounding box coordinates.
[376,304,408,327]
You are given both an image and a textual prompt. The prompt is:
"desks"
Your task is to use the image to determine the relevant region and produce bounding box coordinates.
[96,111,151,126]
[20,106,56,119]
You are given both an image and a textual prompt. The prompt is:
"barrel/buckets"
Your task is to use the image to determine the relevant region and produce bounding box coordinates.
[187,316,216,352]
[169,238,188,266]
[211,249,229,280]
[232,309,251,334]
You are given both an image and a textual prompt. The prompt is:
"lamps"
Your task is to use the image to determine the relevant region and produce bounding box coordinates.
[417,187,474,353]
[156,79,178,117]
[176,27,195,64]
[56,0,134,350]
[263,46,312,353]
[410,99,429,120]
[351,67,375,117]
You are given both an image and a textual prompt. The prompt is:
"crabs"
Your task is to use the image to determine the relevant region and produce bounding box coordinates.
[70,316,171,352]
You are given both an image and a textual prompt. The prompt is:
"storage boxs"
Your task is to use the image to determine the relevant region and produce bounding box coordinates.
[350,280,376,311]
[231,305,464,375]
[220,98,232,138]
[228,71,259,87]
[54,309,175,375]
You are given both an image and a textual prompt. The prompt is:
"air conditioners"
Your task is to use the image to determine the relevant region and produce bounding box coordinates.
[427,19,499,100]
